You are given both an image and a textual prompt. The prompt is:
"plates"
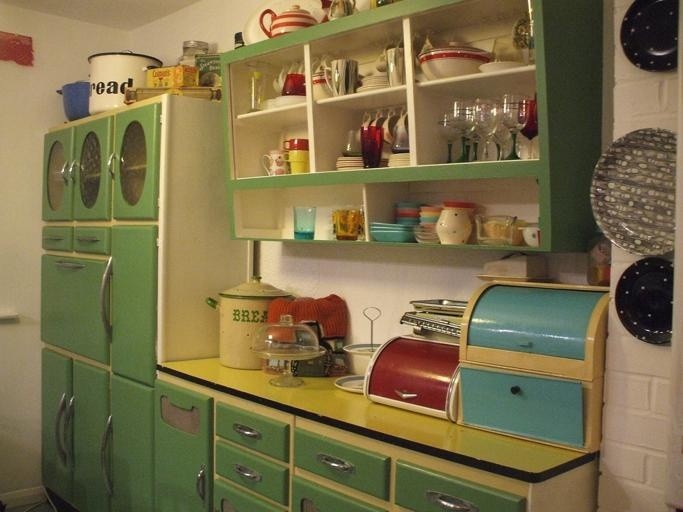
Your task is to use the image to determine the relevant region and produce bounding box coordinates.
[589,127,674,258]
[335,156,365,170]
[614,257,672,347]
[410,298,468,317]
[620,0,677,71]
[387,153,412,167]
[266,95,307,110]
[368,201,441,244]
[442,199,476,210]
[478,61,526,73]
[356,75,389,92]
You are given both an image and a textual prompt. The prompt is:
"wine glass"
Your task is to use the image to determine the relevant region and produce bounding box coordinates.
[438,93,537,163]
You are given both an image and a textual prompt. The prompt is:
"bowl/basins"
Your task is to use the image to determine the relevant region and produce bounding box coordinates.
[472,214,517,244]
[521,228,540,246]
[415,47,492,81]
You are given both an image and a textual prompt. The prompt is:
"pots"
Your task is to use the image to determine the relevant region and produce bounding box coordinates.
[87,52,163,115]
[56,81,89,120]
[204,276,293,370]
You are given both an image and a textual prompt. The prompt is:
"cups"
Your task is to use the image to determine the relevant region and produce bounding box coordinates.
[492,36,523,62]
[359,107,407,144]
[260,137,309,176]
[374,38,405,86]
[272,55,358,100]
[360,126,384,168]
[333,207,364,241]
[292,205,317,241]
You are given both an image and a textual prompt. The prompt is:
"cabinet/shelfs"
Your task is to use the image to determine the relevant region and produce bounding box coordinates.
[153,351,598,510]
[38,90,257,511]
[217,0,611,254]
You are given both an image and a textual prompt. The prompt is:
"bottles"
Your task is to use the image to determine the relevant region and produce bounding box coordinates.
[436,209,472,244]
[341,128,363,157]
[391,124,409,154]
[248,71,264,112]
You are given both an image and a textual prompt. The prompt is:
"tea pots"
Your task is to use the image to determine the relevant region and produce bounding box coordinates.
[260,5,328,39]
[328,0,356,21]
